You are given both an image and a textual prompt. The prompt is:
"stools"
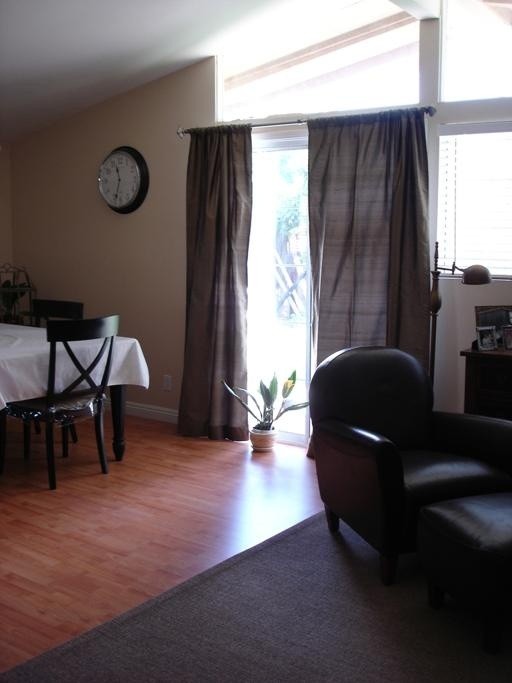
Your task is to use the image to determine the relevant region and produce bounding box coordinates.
[415,491,510,651]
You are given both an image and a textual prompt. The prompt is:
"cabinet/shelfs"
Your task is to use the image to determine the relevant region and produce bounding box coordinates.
[458,330,511,418]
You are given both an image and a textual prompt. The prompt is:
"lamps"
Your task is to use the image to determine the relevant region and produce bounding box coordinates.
[424,239,492,413]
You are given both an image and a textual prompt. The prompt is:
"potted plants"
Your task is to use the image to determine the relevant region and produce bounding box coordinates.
[221,365,311,453]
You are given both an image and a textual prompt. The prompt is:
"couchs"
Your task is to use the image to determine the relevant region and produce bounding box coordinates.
[310,345,511,583]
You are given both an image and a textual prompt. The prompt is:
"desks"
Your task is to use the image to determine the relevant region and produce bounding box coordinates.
[0,320,151,462]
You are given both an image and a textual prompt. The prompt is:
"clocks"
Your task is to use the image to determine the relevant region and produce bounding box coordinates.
[97,145,148,213]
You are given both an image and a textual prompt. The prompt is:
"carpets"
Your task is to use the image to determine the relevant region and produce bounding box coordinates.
[0,505,512,679]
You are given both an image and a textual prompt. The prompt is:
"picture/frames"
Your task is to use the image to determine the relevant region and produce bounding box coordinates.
[474,303,511,326]
[502,326,512,349]
[477,327,496,351]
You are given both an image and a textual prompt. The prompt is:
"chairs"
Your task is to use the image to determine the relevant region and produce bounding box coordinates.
[24,297,85,458]
[7,313,121,490]
